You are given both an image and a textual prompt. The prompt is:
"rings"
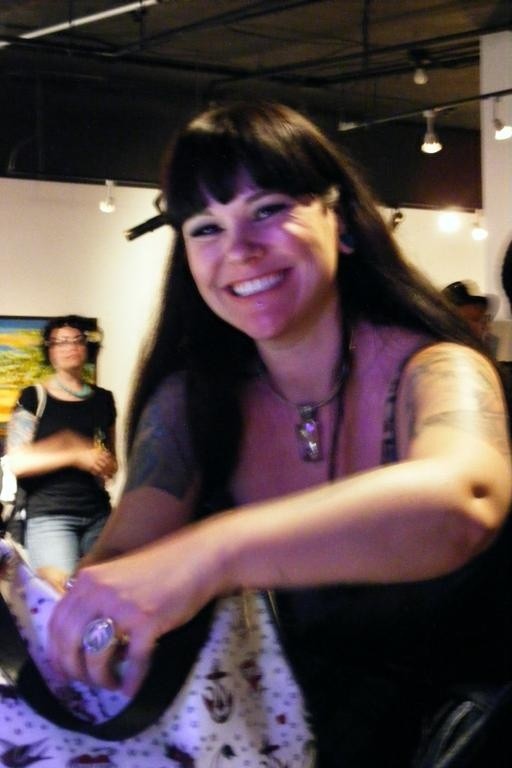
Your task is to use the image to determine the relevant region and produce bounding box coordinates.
[80,613,129,654]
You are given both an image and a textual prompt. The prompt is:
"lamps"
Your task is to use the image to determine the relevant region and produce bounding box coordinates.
[420,97,512,155]
[97,179,118,214]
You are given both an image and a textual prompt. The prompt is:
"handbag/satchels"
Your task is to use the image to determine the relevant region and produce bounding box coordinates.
[0,535,320,767]
[0,455,26,511]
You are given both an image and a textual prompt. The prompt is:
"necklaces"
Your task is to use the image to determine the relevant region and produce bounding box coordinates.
[253,323,359,462]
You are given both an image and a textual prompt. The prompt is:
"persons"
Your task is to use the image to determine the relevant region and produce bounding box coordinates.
[4,313,120,594]
[1,91,512,767]
[495,242,511,403]
[440,281,499,358]
